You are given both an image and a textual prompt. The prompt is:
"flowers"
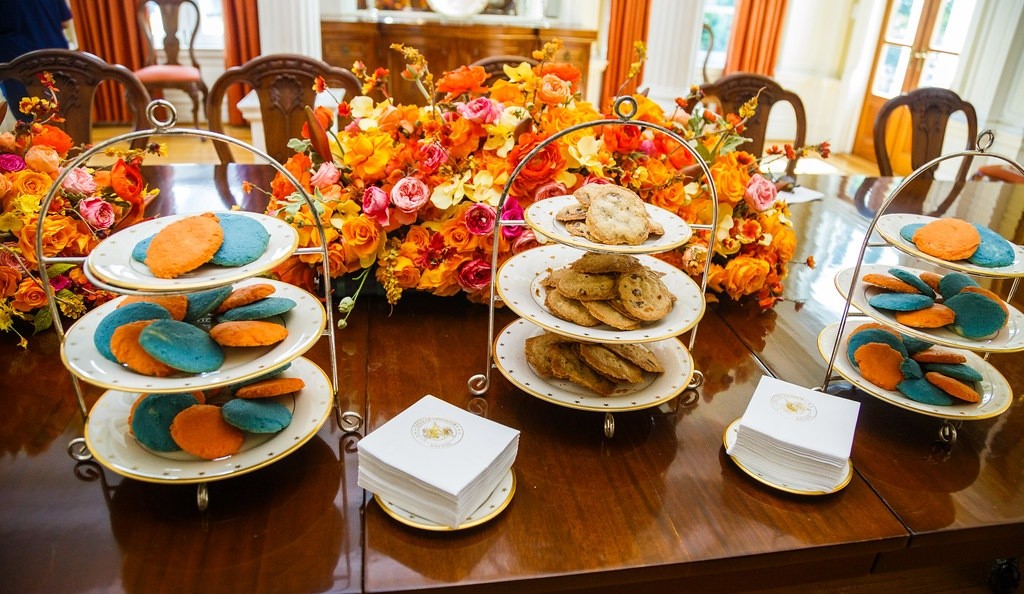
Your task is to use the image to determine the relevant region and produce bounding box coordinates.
[228,36,826,311]
[0,105,142,351]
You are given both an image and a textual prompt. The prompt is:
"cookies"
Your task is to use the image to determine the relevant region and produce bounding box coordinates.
[524,184,677,395]
[93,211,305,461]
[845,216,1015,407]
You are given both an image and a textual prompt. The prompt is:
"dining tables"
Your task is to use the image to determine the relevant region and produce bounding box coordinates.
[0,153,1023,593]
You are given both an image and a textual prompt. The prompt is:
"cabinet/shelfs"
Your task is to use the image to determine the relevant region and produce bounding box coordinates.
[322,9,600,103]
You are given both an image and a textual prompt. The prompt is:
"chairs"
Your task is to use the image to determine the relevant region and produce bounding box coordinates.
[0,48,150,166]
[869,85,979,181]
[685,69,805,153]
[135,1,209,139]
[207,55,363,166]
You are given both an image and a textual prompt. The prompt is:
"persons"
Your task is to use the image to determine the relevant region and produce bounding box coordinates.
[0,0,73,124]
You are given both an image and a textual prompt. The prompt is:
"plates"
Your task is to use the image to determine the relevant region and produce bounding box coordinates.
[374,466,517,531]
[724,417,852,494]
